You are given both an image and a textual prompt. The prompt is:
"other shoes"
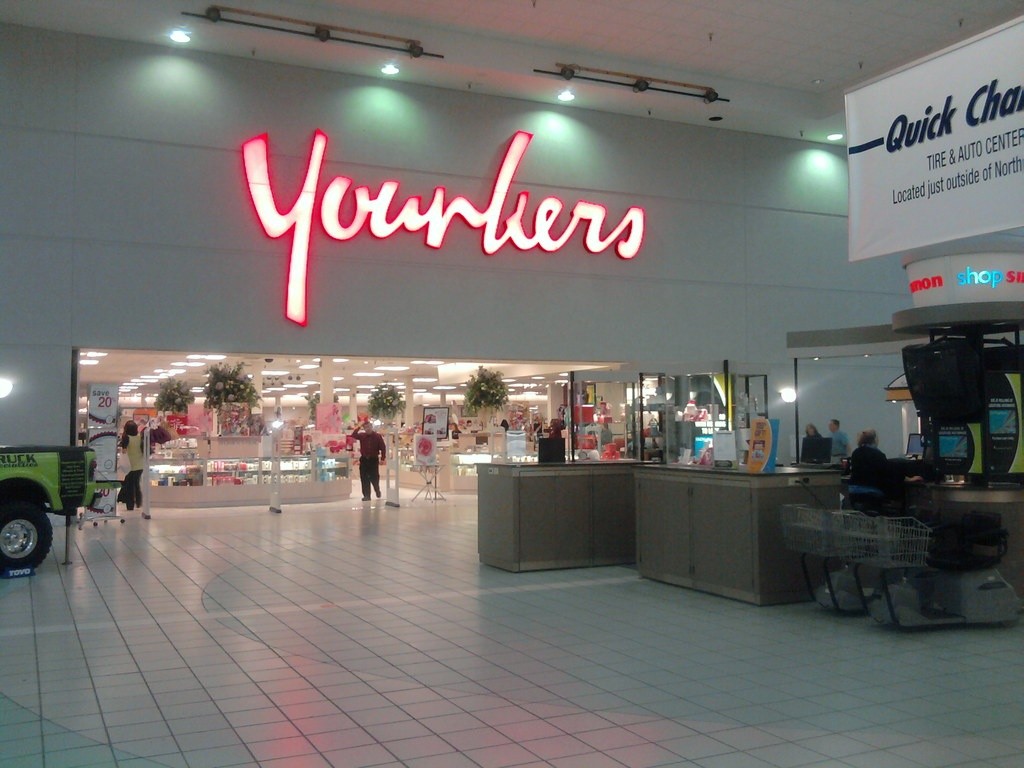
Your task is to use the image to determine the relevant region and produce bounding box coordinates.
[376,491,380,498]
[362,496,371,501]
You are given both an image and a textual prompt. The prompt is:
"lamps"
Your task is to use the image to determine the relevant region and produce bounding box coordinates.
[204,7,224,23]
[404,39,425,57]
[634,77,650,92]
[705,90,718,102]
[561,65,574,80]
[313,27,333,42]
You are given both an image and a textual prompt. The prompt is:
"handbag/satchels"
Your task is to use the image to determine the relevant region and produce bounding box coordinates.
[149,422,171,445]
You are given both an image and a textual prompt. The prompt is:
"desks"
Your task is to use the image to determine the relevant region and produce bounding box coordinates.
[407,464,447,502]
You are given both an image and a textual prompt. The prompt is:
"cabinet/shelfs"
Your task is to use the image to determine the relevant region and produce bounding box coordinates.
[450,453,538,495]
[148,455,351,508]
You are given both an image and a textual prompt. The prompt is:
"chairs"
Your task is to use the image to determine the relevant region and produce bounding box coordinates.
[927,511,1010,569]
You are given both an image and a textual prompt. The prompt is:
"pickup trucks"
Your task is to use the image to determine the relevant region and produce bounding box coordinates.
[0,444,97,578]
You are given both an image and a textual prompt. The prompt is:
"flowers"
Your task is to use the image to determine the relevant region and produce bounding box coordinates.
[465,374,511,410]
[367,381,406,421]
[205,363,261,414]
[154,380,196,416]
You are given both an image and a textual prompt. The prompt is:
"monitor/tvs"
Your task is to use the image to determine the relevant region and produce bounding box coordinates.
[906,433,924,460]
[800,437,832,464]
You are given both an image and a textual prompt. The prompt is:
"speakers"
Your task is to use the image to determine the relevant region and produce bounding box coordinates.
[902,337,990,424]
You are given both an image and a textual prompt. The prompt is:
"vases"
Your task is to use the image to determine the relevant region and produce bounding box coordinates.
[378,404,393,425]
[476,405,492,424]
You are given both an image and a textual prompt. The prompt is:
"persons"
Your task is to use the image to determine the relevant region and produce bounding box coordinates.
[450,423,462,438]
[823,419,850,464]
[848,429,923,487]
[120,421,144,510]
[352,421,386,501]
[806,423,822,437]
